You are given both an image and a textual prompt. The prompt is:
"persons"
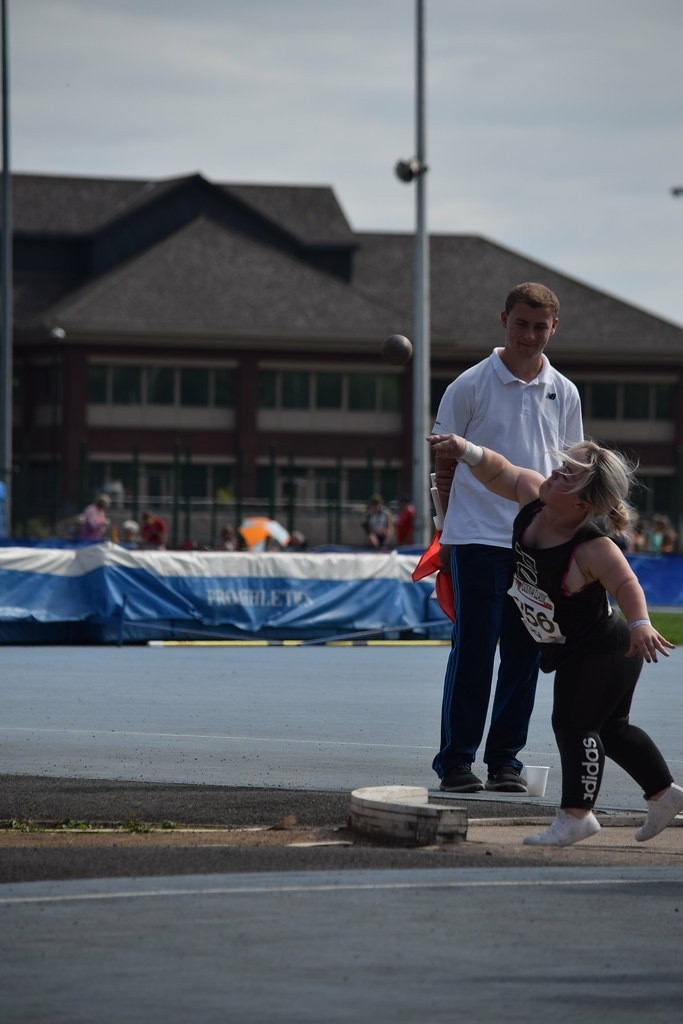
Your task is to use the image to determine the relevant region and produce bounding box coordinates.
[139,513,167,550]
[395,496,415,546]
[119,520,140,550]
[425,434,683,847]
[432,283,584,793]
[76,510,111,551]
[217,523,308,554]
[616,512,678,555]
[360,495,397,550]
[78,494,112,538]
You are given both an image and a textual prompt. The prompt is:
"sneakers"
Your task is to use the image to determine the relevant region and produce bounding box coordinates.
[635,782,683,842]
[522,807,601,846]
[485,766,528,792]
[439,770,485,792]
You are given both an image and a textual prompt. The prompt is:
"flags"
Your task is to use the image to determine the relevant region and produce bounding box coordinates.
[411,528,457,624]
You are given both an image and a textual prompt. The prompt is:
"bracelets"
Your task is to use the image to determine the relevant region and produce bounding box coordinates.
[458,440,483,467]
[628,621,650,631]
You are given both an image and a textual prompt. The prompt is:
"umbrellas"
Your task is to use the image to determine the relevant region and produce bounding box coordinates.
[239,517,291,552]
